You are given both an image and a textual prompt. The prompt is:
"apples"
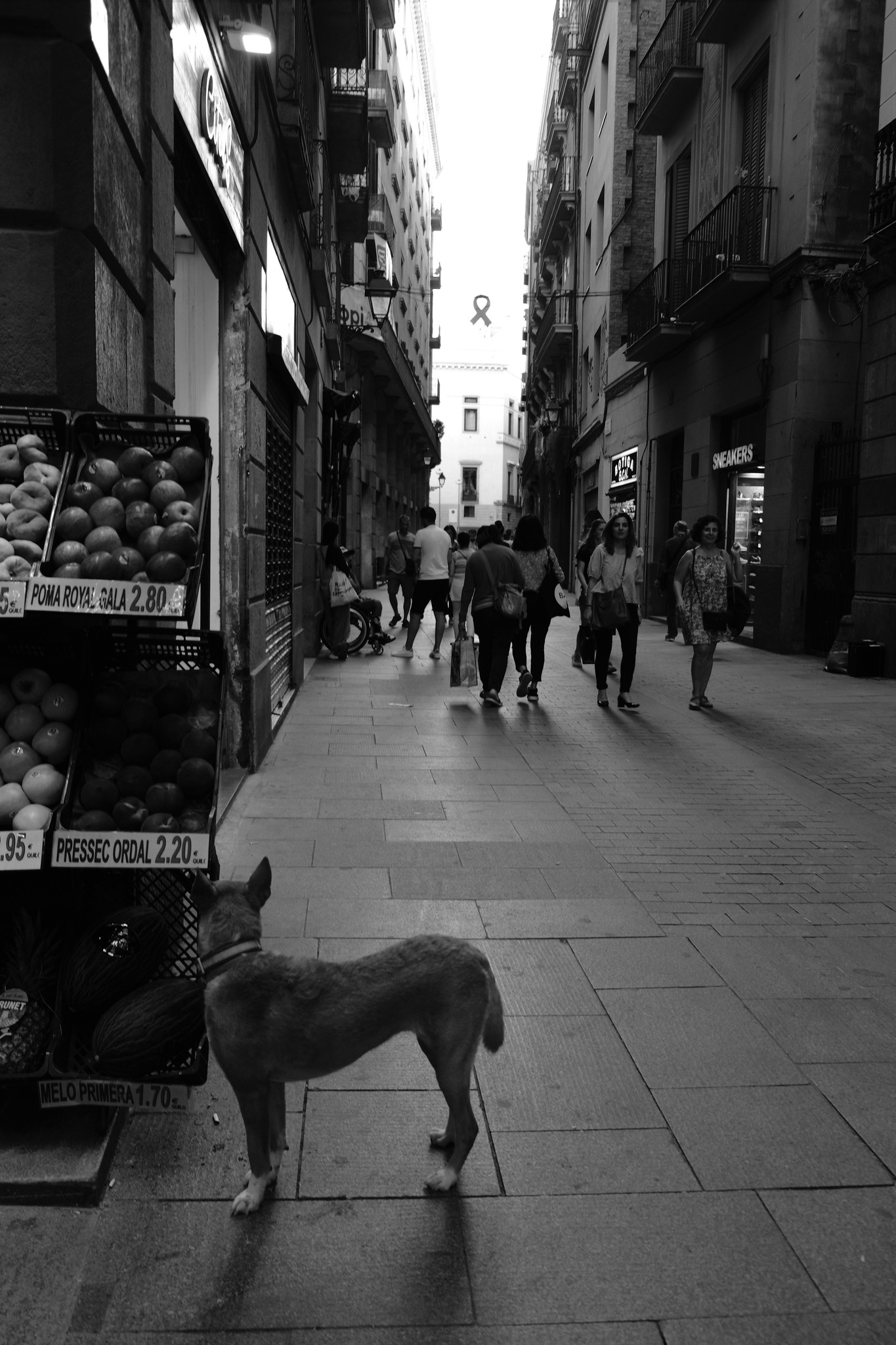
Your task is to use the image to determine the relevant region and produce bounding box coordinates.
[0,668,77,831]
[53,447,203,583]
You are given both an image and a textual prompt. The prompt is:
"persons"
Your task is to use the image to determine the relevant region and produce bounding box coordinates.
[673,514,744,711]
[340,546,396,646]
[582,512,645,708]
[391,506,452,659]
[444,521,517,622]
[654,521,697,646]
[571,518,617,673]
[456,524,525,707]
[512,514,565,701]
[384,514,415,628]
[318,521,351,659]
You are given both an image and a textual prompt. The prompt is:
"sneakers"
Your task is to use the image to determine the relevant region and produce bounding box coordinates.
[607,661,617,673]
[571,653,582,668]
[516,668,532,698]
[429,648,441,660]
[391,645,413,658]
[527,686,539,701]
[732,491,764,564]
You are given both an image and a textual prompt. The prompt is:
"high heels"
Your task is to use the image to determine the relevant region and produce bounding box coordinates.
[597,693,609,707]
[617,694,640,707]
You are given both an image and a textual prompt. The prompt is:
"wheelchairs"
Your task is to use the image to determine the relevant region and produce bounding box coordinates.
[320,608,395,656]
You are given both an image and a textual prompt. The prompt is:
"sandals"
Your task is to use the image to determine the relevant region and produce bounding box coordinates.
[389,614,402,626]
[368,632,396,644]
[401,619,409,628]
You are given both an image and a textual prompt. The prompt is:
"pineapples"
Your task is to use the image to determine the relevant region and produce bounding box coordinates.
[0,999,50,1069]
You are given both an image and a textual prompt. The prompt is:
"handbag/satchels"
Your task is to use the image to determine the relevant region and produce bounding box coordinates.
[702,610,728,632]
[587,584,630,633]
[494,582,528,619]
[405,558,419,575]
[658,570,669,591]
[726,584,752,638]
[578,623,595,665]
[450,627,478,688]
[330,565,360,607]
[536,561,570,620]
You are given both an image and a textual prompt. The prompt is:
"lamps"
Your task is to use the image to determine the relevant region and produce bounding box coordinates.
[342,269,397,342]
[429,472,446,492]
[545,397,577,435]
[219,15,274,55]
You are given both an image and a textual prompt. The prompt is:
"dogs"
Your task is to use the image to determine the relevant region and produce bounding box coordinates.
[189,856,505,1217]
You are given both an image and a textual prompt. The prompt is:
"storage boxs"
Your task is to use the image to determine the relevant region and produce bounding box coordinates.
[0,406,232,1113]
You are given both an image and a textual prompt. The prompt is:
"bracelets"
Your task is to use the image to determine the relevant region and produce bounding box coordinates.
[637,605,642,609]
[586,605,592,608]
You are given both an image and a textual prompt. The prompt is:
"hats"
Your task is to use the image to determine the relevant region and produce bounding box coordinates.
[340,546,355,556]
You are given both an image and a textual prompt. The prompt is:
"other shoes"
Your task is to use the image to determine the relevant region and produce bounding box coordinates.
[329,643,350,661]
[689,695,714,710]
[665,635,675,641]
[449,619,453,625]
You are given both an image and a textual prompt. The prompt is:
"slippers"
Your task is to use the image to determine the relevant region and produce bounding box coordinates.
[480,689,502,706]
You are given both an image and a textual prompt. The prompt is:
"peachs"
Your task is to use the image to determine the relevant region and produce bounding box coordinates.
[68,704,219,833]
[0,434,60,583]
